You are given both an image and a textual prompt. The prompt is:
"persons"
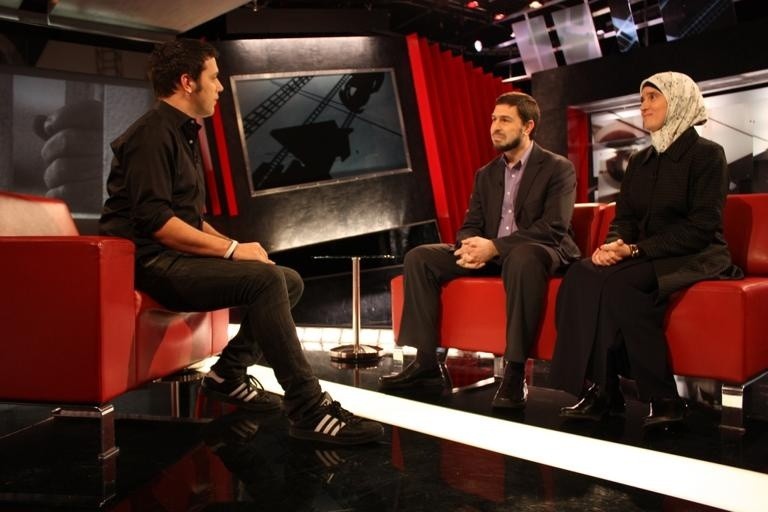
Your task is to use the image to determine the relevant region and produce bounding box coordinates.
[33,99,104,214]
[377,91,582,410]
[551,71,744,434]
[95,36,384,445]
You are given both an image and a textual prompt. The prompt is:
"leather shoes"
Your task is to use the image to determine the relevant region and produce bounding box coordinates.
[378,362,443,386]
[560,383,625,419]
[644,395,690,425]
[493,371,528,408]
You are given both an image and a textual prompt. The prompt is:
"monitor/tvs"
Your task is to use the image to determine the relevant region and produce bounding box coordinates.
[0,63,157,221]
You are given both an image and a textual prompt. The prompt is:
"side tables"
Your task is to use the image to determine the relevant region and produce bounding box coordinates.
[313,255,396,359]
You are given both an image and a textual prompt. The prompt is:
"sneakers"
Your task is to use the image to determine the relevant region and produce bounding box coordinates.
[289,392,384,445]
[204,412,283,440]
[291,445,381,472]
[202,374,281,411]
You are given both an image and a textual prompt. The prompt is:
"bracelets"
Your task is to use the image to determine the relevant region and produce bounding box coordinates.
[629,243,640,257]
[224,240,238,259]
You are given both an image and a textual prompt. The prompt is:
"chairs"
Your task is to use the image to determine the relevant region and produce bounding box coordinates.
[0,190,230,463]
[391,202,606,385]
[595,192,768,438]
